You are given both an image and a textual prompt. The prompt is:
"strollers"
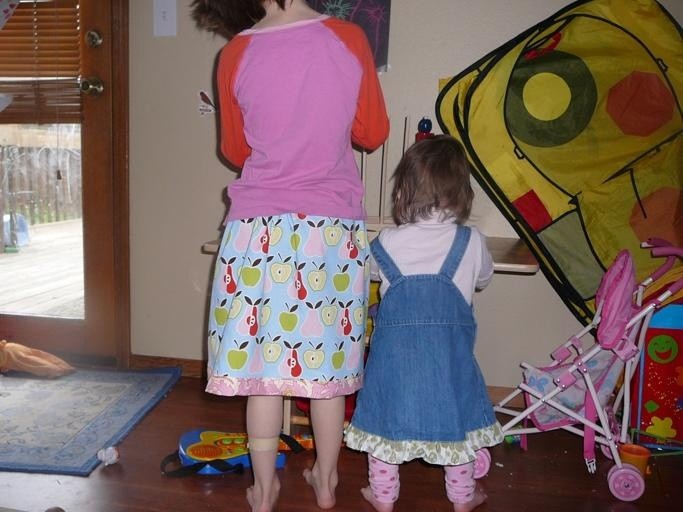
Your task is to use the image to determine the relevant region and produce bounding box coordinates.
[472,245,682,501]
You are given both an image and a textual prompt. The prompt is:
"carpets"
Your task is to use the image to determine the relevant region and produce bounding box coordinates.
[1,362,183,477]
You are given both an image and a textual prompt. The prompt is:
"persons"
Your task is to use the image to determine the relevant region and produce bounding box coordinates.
[189,0,390,511]
[342,133,506,511]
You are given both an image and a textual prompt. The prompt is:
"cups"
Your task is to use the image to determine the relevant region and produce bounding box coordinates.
[621,444,649,479]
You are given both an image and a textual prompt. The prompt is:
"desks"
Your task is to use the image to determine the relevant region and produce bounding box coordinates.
[202,233,540,436]
